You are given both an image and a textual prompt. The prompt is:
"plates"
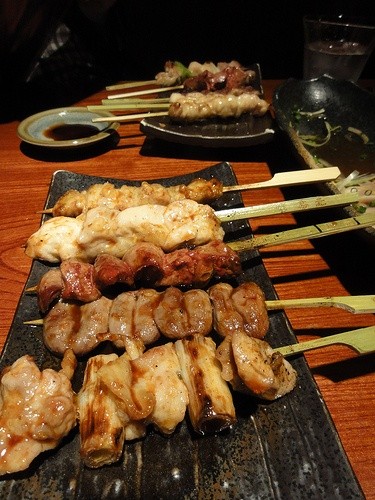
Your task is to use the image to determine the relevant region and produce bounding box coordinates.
[141,62,276,149]
[0,170,369,500]
[272,73,375,245]
[16,107,120,148]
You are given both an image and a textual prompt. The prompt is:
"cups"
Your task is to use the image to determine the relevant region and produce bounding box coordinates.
[303,14,375,84]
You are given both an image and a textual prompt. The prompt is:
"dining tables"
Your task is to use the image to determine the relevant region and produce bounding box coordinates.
[1,81,375,499]
